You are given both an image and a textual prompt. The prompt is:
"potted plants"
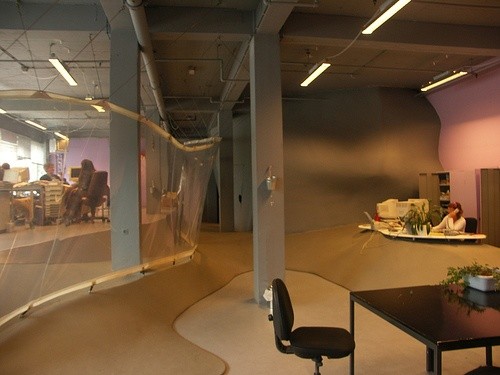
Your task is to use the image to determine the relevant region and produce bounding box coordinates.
[396,203,441,236]
[441,286,500,318]
[434,259,500,292]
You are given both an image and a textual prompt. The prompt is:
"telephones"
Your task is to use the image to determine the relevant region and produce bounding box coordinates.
[443,209,459,236]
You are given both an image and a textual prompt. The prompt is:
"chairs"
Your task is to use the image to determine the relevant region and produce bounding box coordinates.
[268,278,355,374]
[464,217,478,242]
[76,171,111,224]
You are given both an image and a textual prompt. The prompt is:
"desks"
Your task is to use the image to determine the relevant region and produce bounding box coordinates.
[349,282,500,374]
[0,184,72,233]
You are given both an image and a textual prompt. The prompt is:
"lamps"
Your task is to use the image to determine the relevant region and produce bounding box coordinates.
[419,68,468,92]
[300,56,331,87]
[85,98,105,113]
[49,52,78,86]
[54,131,69,141]
[0,108,7,114]
[25,119,47,131]
[361,0,411,35]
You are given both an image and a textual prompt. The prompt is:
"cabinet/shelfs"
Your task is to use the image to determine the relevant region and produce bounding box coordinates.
[417,170,464,221]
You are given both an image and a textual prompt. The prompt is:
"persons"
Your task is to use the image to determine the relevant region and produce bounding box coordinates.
[40,162,62,181]
[432,201,467,234]
[60,159,109,225]
[0,163,10,180]
